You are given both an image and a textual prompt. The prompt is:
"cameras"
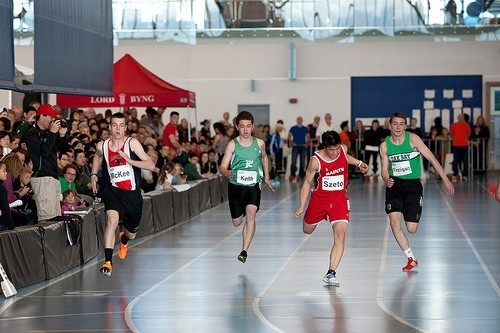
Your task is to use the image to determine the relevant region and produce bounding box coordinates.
[54,117,66,127]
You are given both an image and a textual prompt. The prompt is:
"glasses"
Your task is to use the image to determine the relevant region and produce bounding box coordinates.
[67,173,77,177]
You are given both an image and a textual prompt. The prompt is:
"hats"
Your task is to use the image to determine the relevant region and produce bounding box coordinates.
[37,104,60,118]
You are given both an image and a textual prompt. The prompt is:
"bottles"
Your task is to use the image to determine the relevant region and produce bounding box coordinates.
[93,197,98,206]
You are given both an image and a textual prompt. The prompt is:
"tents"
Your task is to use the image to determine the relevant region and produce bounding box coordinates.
[57,54,199,142]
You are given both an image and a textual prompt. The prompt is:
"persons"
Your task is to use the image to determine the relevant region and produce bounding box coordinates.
[90,112,156,279]
[494,176,500,202]
[219,110,275,263]
[380,112,456,272]
[294,130,369,287]
[0,101,490,231]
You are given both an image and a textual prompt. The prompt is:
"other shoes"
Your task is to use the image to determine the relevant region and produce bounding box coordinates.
[297,174,301,182]
[364,173,371,182]
[461,176,468,181]
[289,175,294,181]
[452,175,456,182]
[372,173,378,180]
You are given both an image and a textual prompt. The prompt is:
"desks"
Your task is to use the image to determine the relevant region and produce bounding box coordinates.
[0,174,231,294]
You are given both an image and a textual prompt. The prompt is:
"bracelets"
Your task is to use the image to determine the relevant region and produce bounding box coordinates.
[90,173,98,177]
[356,160,362,168]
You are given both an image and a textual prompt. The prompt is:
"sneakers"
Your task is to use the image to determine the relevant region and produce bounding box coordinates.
[401,256,419,271]
[118,232,128,259]
[322,273,340,286]
[100,261,113,276]
[237,250,248,264]
